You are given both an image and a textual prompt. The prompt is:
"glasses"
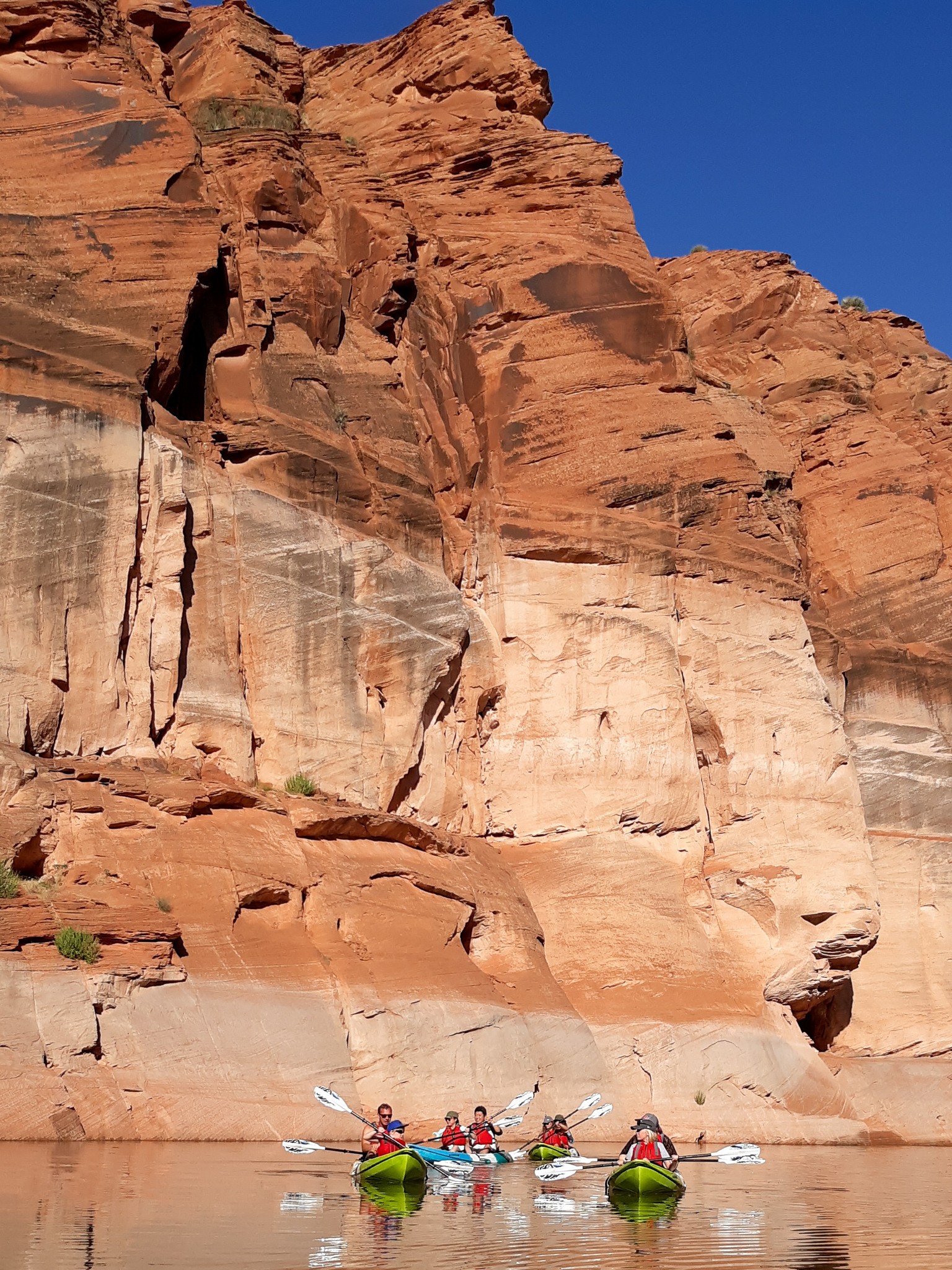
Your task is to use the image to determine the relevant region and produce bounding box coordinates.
[379,1114,392,1118]
[445,1118,453,1122]
[395,1130,404,1134]
[543,1122,550,1125]
[637,1120,652,1127]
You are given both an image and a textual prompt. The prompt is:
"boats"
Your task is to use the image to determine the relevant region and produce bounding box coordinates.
[348,1147,429,1184]
[350,1175,430,1219]
[528,1142,572,1161]
[407,1142,525,1168]
[604,1158,686,1194]
[606,1191,684,1228]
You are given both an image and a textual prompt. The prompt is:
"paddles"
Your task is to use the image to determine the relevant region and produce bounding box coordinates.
[406,1113,524,1145]
[533,1143,761,1181]
[553,1155,766,1165]
[510,1102,614,1160]
[314,1085,467,1182]
[467,1090,535,1131]
[507,1092,601,1156]
[280,1138,474,1176]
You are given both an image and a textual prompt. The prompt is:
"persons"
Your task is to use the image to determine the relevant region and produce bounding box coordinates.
[362,1103,410,1162]
[423,1105,503,1154]
[535,1114,574,1150]
[617,1113,679,1172]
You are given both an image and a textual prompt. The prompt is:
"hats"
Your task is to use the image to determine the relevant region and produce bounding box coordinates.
[630,1120,655,1132]
[387,1120,409,1132]
[445,1111,459,1118]
[542,1116,553,1124]
[635,1113,658,1128]
[554,1115,566,1123]
